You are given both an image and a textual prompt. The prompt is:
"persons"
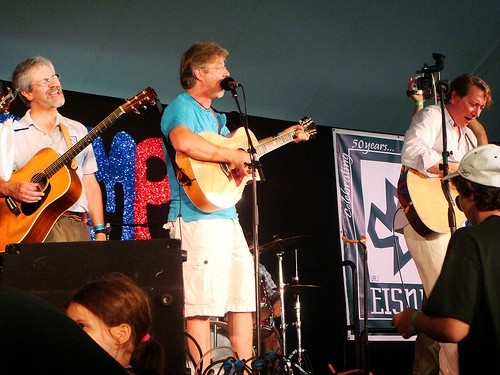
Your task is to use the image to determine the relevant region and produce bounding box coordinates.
[392,73,500,375]
[64,271,166,375]
[160,40,310,375]
[0,284,128,375]
[241,228,286,358]
[0,56,112,242]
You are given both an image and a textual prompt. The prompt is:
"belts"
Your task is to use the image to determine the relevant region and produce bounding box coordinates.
[63,210,84,221]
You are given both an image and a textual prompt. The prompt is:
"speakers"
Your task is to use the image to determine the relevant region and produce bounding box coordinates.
[0,239,187,375]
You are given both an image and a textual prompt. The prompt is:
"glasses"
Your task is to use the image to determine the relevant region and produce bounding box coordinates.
[28,73,61,89]
[198,64,227,73]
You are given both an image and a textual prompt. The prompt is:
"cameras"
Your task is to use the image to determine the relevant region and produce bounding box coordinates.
[412,71,433,94]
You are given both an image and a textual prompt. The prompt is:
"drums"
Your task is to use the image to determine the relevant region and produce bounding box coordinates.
[251,272,276,341]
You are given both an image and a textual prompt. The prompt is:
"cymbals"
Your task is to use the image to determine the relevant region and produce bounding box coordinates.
[249,233,310,256]
[285,283,320,292]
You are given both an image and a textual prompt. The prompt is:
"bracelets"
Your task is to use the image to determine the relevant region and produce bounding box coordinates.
[93,223,111,236]
[414,100,425,106]
[273,132,283,138]
[411,309,420,332]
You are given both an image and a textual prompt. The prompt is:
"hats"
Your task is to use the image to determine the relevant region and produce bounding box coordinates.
[441,144,500,188]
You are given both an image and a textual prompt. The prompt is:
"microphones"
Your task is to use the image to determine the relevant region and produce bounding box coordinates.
[221,77,240,91]
[435,80,449,94]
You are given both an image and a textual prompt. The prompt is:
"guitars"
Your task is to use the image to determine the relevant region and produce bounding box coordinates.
[175,115,317,214]
[397,168,469,240]
[0,86,157,254]
